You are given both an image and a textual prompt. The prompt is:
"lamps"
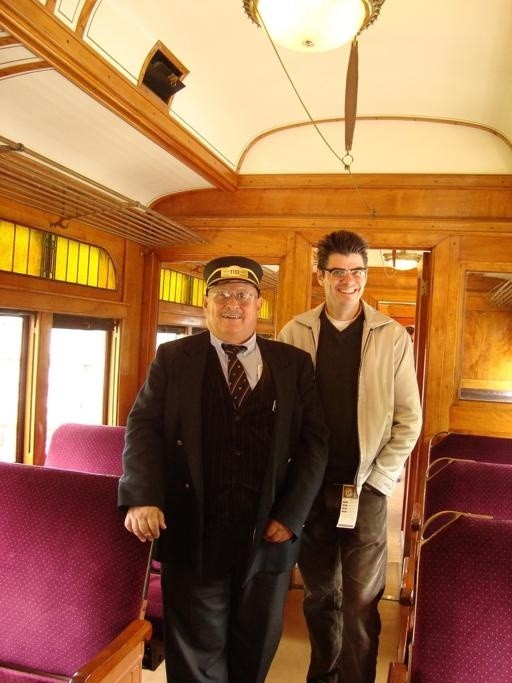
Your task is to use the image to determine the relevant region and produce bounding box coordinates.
[243,0,383,52]
[383,250,422,270]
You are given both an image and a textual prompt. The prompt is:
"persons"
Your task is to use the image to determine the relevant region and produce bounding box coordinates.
[115,256,332,682]
[277,230,424,682]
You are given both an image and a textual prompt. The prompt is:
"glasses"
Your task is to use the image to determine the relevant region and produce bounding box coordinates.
[212,285,255,307]
[323,267,368,279]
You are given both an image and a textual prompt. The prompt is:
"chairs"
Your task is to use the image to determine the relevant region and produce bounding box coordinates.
[0,461,154,683]
[411,431,512,553]
[397,458,512,662]
[44,423,163,670]
[387,510,512,682]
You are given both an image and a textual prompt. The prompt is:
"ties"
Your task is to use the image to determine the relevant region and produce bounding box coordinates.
[221,343,252,409]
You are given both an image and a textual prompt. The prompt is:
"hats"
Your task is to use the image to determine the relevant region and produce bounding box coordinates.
[203,255,264,291]
